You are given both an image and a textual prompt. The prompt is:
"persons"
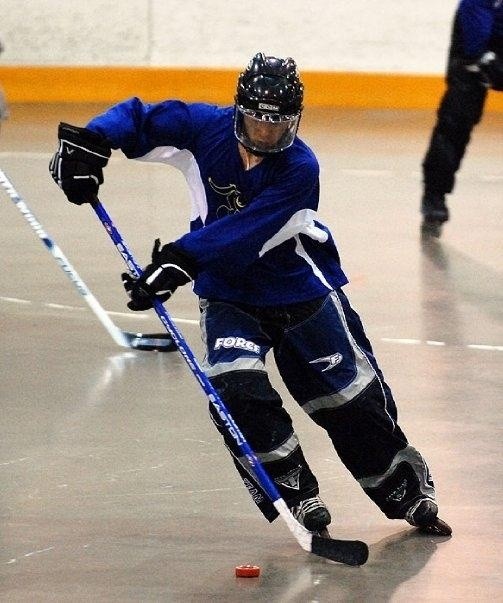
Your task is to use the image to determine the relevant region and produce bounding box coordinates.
[49,52,438,528]
[420,0,503,226]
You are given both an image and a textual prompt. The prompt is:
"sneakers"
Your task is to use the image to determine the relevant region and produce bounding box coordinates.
[420,185,452,224]
[290,495,331,532]
[403,496,439,529]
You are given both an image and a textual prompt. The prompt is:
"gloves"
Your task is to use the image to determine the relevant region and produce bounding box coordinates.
[118,235,196,312]
[47,121,113,205]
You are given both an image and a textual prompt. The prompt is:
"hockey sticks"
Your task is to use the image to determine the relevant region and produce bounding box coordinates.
[0,170,180,351]
[90,193,368,566]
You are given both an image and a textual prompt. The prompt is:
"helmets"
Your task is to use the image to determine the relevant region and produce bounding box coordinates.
[233,52,305,125]
